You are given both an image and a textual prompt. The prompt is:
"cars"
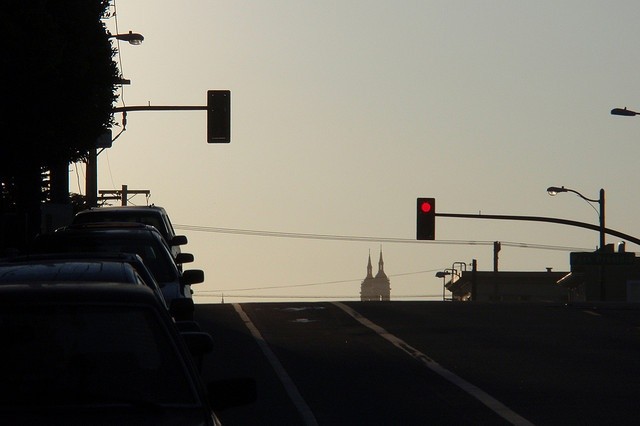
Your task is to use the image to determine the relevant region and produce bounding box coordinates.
[68,222,194,269]
[0,259,202,341]
[3,250,195,320]
[1,283,215,425]
[72,205,188,247]
[17,227,204,301]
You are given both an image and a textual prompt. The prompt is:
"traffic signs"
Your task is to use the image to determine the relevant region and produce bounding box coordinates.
[608,106,640,123]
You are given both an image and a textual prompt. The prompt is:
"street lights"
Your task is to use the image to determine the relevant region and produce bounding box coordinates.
[547,186,608,301]
[86,31,144,203]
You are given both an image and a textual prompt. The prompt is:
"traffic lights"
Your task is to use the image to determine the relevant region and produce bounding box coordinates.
[416,197,435,240]
